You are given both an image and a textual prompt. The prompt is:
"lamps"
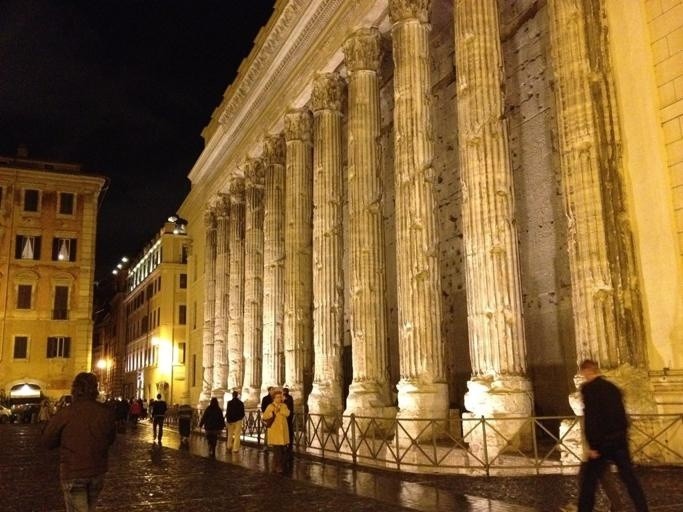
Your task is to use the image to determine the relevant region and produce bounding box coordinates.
[168,214,188,226]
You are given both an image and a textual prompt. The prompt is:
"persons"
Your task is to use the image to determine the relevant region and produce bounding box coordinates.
[37,371,116,511]
[574,356,647,512]
[38,384,293,469]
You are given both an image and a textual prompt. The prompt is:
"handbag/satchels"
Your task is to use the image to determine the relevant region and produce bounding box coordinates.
[261,403,276,428]
